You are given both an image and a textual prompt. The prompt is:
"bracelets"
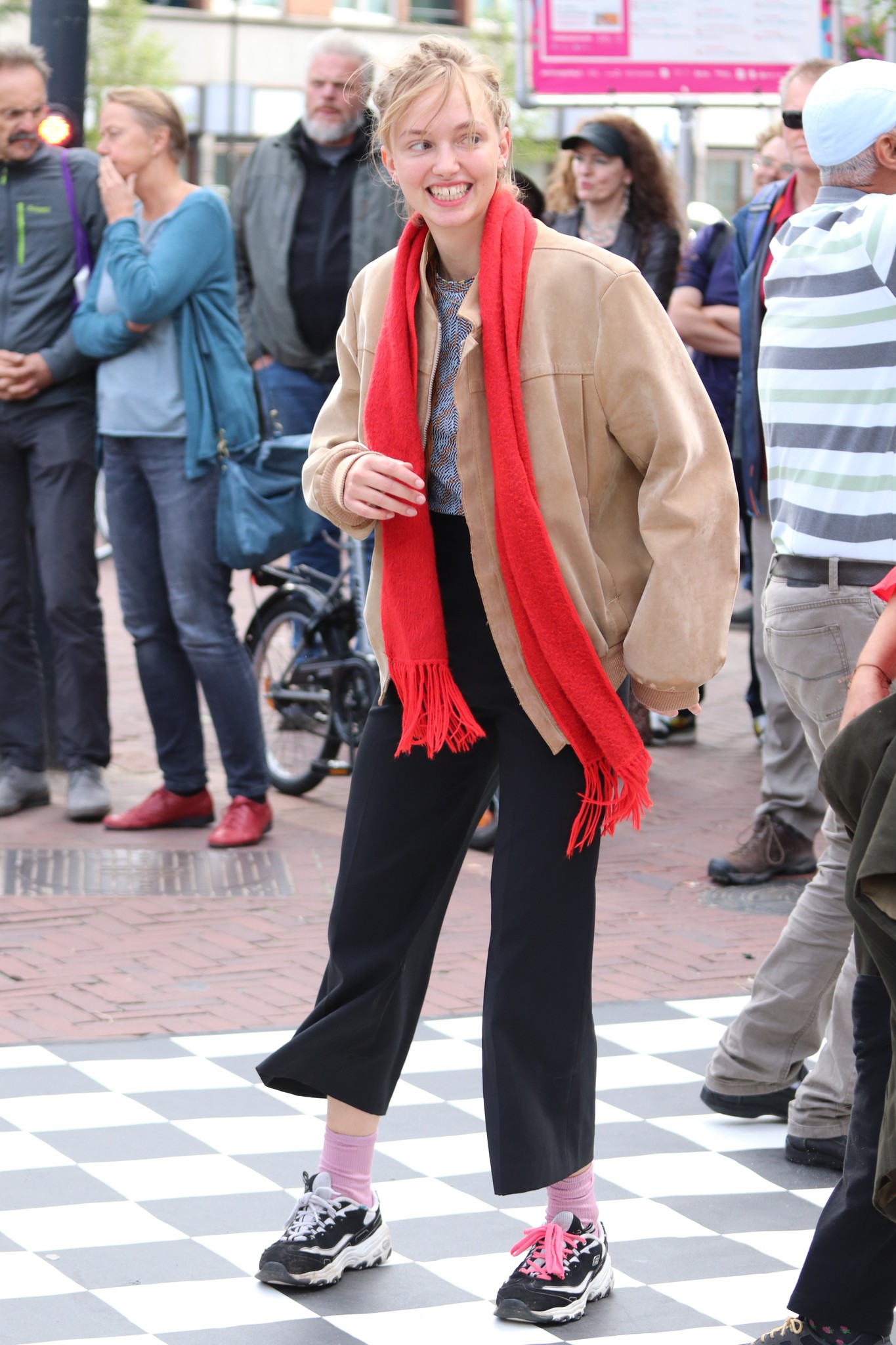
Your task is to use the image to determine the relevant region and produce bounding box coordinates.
[836,666,893,691]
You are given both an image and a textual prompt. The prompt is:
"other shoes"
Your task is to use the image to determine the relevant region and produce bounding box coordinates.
[649,712,695,744]
[752,715,767,743]
[698,1062,808,1120]
[784,1134,847,1170]
[742,1315,892,1345]
[66,767,112,821]
[0,765,50,815]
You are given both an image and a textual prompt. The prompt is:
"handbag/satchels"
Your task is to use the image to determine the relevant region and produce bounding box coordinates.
[214,440,328,568]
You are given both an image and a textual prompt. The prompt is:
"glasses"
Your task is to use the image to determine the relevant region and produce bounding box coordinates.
[781,111,802,128]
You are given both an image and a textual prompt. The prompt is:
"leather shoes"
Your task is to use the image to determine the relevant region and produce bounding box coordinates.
[103,784,214,831]
[208,793,274,847]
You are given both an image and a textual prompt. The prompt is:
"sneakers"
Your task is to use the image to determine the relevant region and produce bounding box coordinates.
[708,813,817,884]
[254,1171,393,1287]
[494,1211,614,1325]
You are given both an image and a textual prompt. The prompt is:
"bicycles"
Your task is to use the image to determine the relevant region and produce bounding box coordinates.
[235,536,505,852]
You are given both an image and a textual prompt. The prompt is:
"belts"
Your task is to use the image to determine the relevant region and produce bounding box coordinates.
[769,554,895,585]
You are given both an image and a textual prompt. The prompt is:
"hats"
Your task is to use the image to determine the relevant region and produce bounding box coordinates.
[801,59,896,166]
[562,125,630,167]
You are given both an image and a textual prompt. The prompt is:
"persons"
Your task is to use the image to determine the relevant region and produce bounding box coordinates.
[0,29,896,1345]
